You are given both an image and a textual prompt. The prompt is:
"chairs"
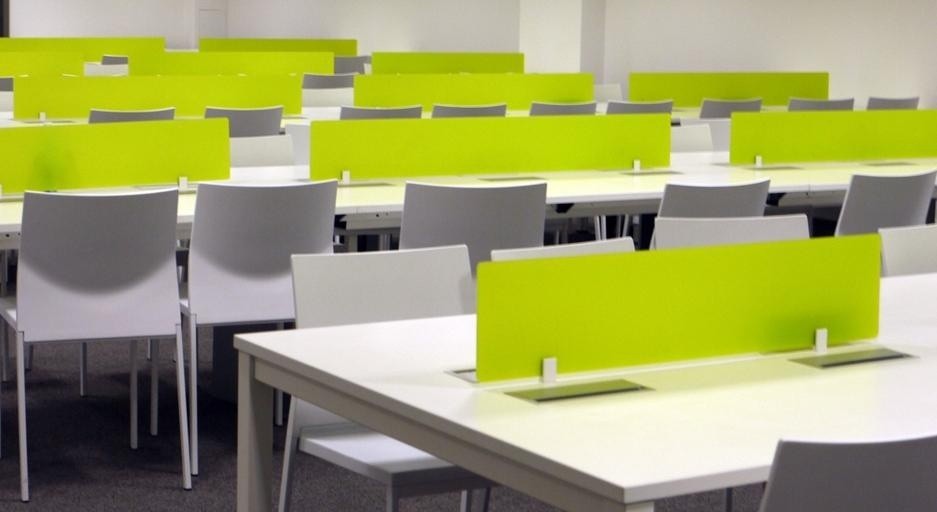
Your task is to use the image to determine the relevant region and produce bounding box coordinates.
[655,214,808,250]
[303,87,355,107]
[529,103,597,116]
[700,99,761,118]
[0,189,192,503]
[758,438,936,510]
[492,236,636,263]
[865,97,919,109]
[277,244,496,510]
[680,117,730,150]
[230,135,295,168]
[102,56,128,66]
[0,78,14,90]
[878,223,937,277]
[787,98,854,111]
[0,91,14,113]
[84,63,130,76]
[149,177,338,477]
[340,106,422,119]
[835,168,937,235]
[286,123,310,164]
[204,106,283,136]
[334,55,371,74]
[88,107,176,123]
[431,105,507,119]
[670,123,712,152]
[303,73,357,88]
[648,180,770,249]
[606,102,672,113]
[398,183,546,270]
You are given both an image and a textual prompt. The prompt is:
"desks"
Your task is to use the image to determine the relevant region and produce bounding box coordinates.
[0,164,937,283]
[232,272,937,511]
